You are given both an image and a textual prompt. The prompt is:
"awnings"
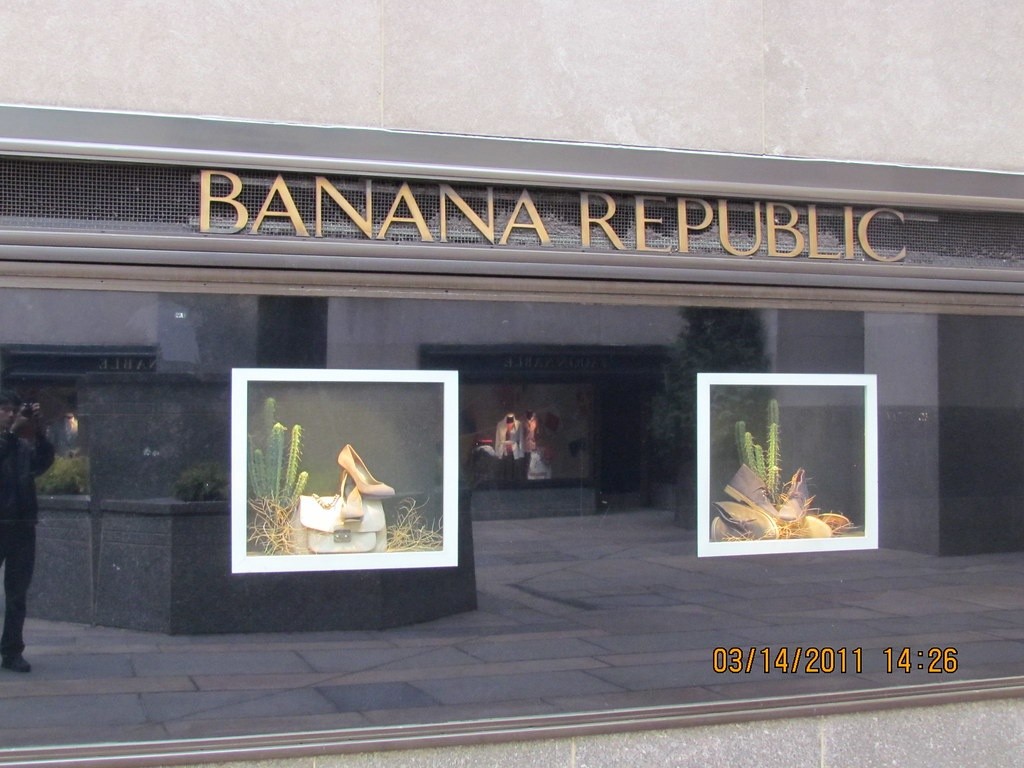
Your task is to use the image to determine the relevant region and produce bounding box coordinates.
[419,344,683,392]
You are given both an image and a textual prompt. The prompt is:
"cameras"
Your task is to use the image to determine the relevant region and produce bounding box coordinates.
[20,404,34,418]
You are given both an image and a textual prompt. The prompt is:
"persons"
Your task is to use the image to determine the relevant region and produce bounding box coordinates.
[494,413,525,480]
[0,389,55,672]
[522,409,561,479]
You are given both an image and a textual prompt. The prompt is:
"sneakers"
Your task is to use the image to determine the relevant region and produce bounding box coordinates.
[711,464,851,542]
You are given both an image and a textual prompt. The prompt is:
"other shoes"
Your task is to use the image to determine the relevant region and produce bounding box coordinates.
[2,654,30,672]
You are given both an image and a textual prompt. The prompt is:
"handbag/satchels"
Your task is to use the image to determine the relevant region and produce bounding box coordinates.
[288,494,387,554]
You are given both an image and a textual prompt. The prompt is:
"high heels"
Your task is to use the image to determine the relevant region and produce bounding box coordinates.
[338,444,395,496]
